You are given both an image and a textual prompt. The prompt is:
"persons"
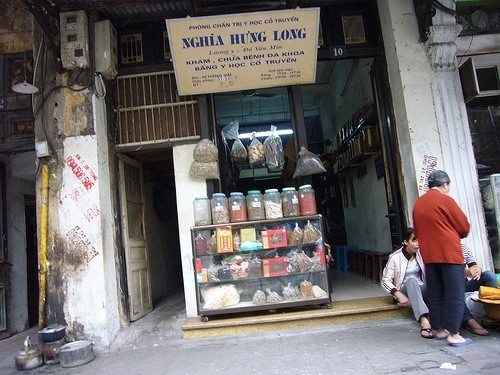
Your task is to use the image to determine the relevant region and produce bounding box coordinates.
[461,244,498,337]
[412,171,472,346]
[381,228,436,339]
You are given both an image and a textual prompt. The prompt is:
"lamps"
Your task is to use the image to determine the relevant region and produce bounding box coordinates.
[10,11,39,93]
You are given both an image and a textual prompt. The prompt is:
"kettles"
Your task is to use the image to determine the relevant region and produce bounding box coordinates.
[15,336,44,370]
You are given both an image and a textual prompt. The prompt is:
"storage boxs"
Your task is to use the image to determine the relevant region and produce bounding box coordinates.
[196,226,288,283]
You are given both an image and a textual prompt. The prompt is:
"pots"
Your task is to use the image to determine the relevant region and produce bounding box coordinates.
[37,323,66,341]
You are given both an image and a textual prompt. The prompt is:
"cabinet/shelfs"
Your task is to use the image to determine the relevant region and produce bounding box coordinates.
[478,172,500,271]
[190,214,333,321]
[337,111,380,174]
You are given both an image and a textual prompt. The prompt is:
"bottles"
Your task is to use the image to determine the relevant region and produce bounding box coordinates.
[246,189,266,222]
[281,187,300,218]
[228,191,249,222]
[210,192,230,224]
[298,183,318,216]
[193,197,213,227]
[264,188,284,221]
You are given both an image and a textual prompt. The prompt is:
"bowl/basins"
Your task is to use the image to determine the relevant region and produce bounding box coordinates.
[54,339,96,368]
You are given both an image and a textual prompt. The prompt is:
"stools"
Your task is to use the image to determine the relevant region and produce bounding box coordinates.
[330,244,393,284]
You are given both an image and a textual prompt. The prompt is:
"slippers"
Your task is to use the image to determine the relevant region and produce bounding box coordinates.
[435,335,447,340]
[421,329,435,339]
[450,337,473,347]
[464,325,490,336]
